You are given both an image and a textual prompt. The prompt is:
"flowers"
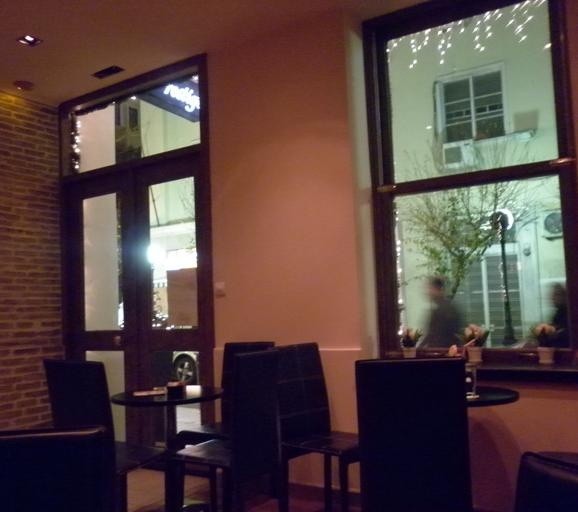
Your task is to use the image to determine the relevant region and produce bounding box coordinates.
[528,322,565,346]
[399,326,423,346]
[454,325,490,346]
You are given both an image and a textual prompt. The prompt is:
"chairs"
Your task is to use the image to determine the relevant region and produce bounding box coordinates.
[163,341,275,509]
[353,357,474,509]
[41,357,163,509]
[384,343,577,387]
[1,422,121,509]
[235,343,360,511]
[510,451,576,510]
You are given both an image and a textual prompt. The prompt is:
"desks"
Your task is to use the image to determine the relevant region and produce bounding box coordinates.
[110,384,224,510]
[464,387,522,408]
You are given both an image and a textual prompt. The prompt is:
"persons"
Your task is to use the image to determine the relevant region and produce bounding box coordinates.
[416,277,466,348]
[529,279,573,348]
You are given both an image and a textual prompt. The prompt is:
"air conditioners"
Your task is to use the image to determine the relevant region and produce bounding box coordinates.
[442,139,477,169]
